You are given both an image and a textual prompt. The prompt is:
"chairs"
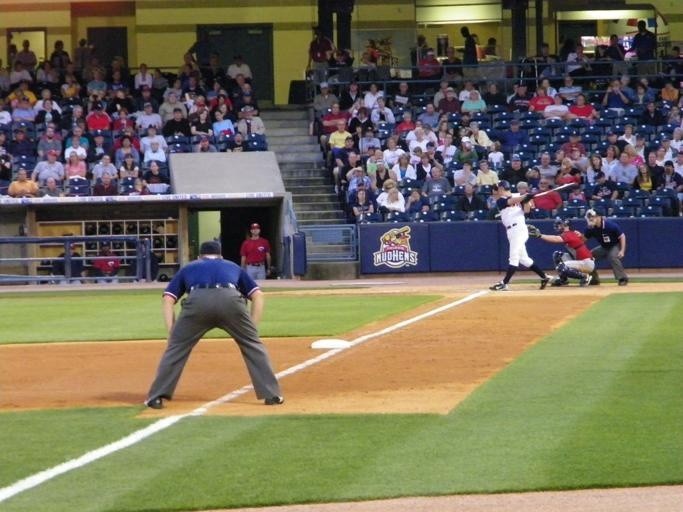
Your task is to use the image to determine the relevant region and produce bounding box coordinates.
[0,96,268,196]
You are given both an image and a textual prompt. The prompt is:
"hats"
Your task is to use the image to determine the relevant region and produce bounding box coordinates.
[497,180,511,189]
[512,156,521,162]
[593,171,605,179]
[461,136,472,143]
[320,81,328,88]
[250,223,261,230]
[144,101,152,108]
[665,159,675,168]
[142,84,152,91]
[199,240,223,255]
[375,158,386,166]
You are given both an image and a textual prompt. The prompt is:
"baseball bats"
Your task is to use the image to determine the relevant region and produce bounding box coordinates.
[532,181,578,198]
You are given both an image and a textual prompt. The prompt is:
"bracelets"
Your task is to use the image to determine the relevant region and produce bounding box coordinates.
[267,268,273,271]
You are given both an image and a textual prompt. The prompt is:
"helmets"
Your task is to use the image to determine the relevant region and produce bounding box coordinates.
[65,240,74,250]
[86,225,178,264]
[585,207,598,227]
[159,273,169,281]
[553,215,569,232]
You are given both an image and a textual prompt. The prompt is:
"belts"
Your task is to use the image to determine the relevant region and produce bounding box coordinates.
[188,282,238,289]
[246,262,266,267]
[505,222,518,230]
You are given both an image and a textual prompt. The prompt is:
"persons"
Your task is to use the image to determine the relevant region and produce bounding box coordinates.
[1,33,268,197]
[49,244,85,285]
[526,218,597,287]
[583,208,630,287]
[93,241,121,284]
[303,20,681,222]
[142,240,286,409]
[129,240,160,283]
[241,223,273,282]
[488,180,552,291]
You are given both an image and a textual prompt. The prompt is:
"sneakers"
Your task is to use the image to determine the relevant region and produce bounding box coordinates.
[265,396,283,405]
[144,397,162,409]
[589,280,601,285]
[489,281,510,291]
[618,278,630,285]
[552,279,570,288]
[580,273,591,287]
[539,272,552,290]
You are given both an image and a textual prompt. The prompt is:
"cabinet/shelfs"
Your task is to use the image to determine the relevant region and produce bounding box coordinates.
[37,219,180,267]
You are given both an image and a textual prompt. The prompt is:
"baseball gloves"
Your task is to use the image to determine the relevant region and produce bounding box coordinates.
[528,224,541,240]
